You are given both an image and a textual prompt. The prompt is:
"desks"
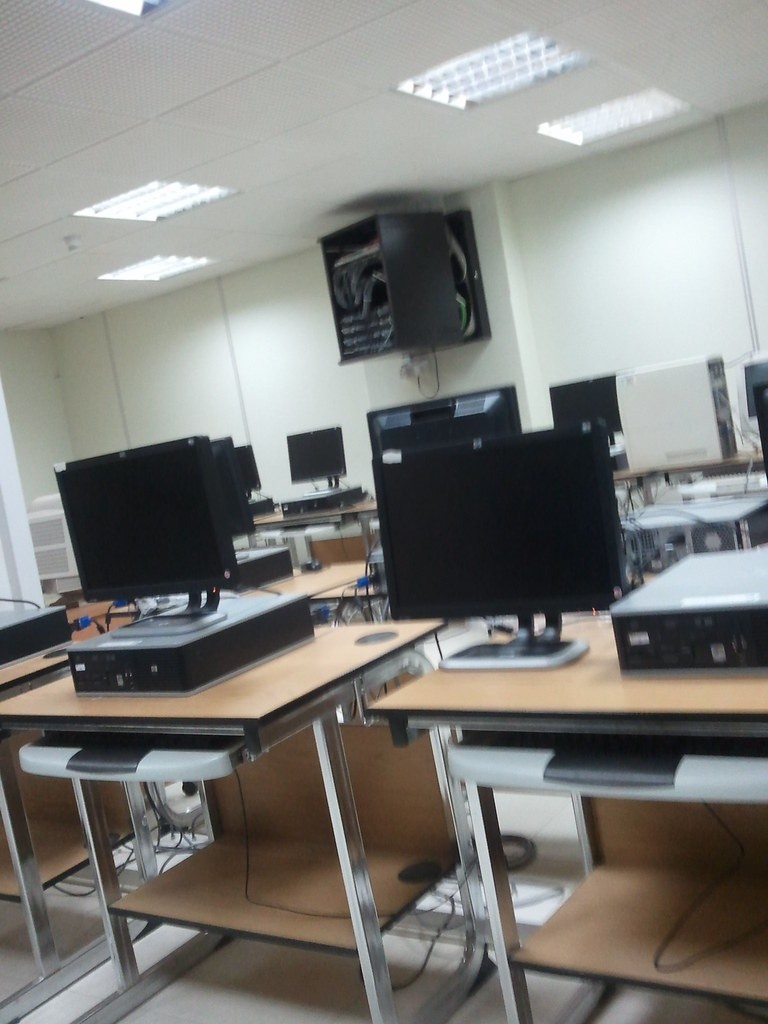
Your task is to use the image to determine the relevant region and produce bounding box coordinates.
[0,434,768,1024]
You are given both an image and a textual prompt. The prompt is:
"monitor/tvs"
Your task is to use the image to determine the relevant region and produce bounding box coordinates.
[49,354,741,672]
